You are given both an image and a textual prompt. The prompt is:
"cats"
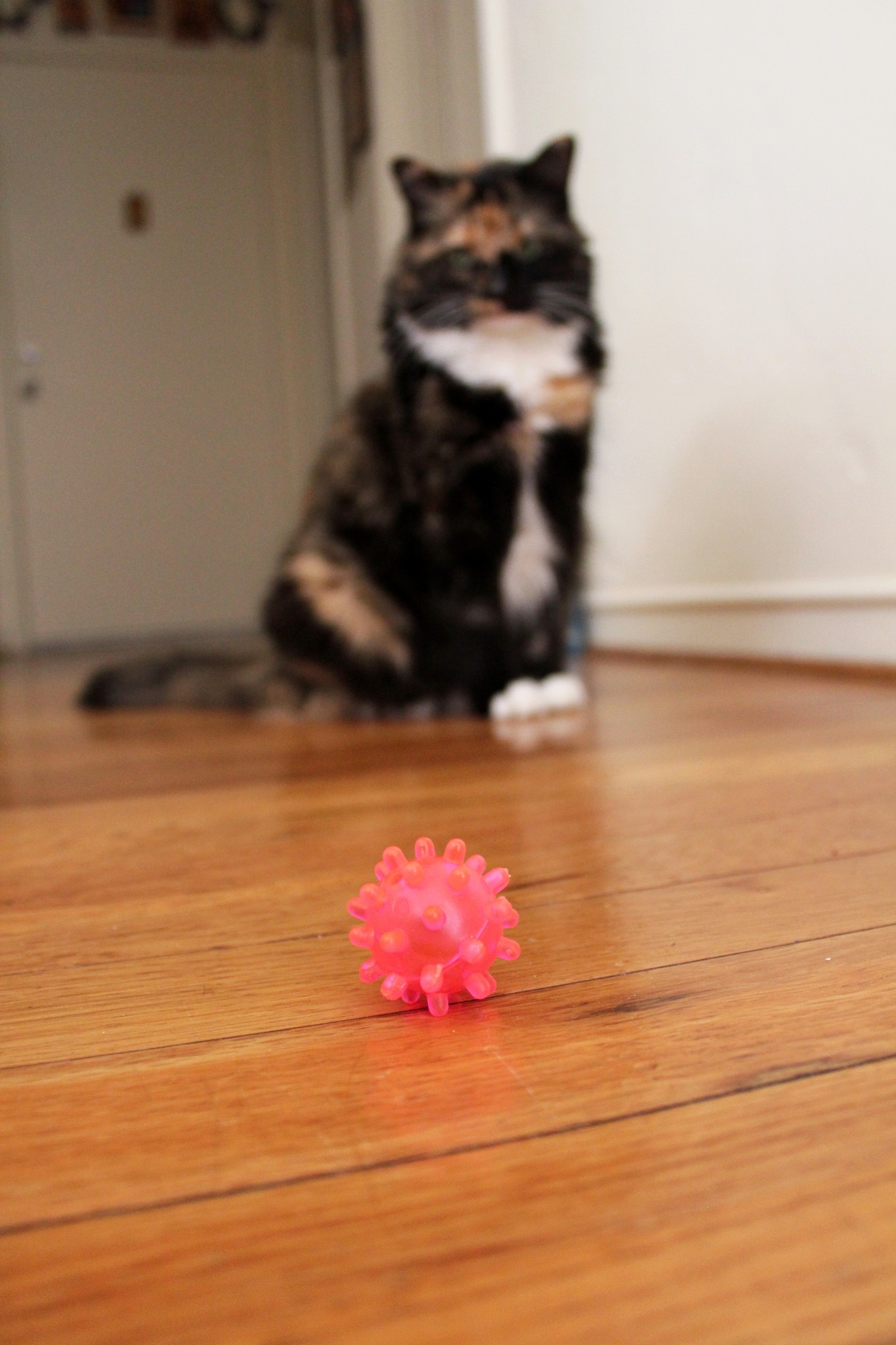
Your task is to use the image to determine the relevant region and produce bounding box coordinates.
[72,135,623,723]
